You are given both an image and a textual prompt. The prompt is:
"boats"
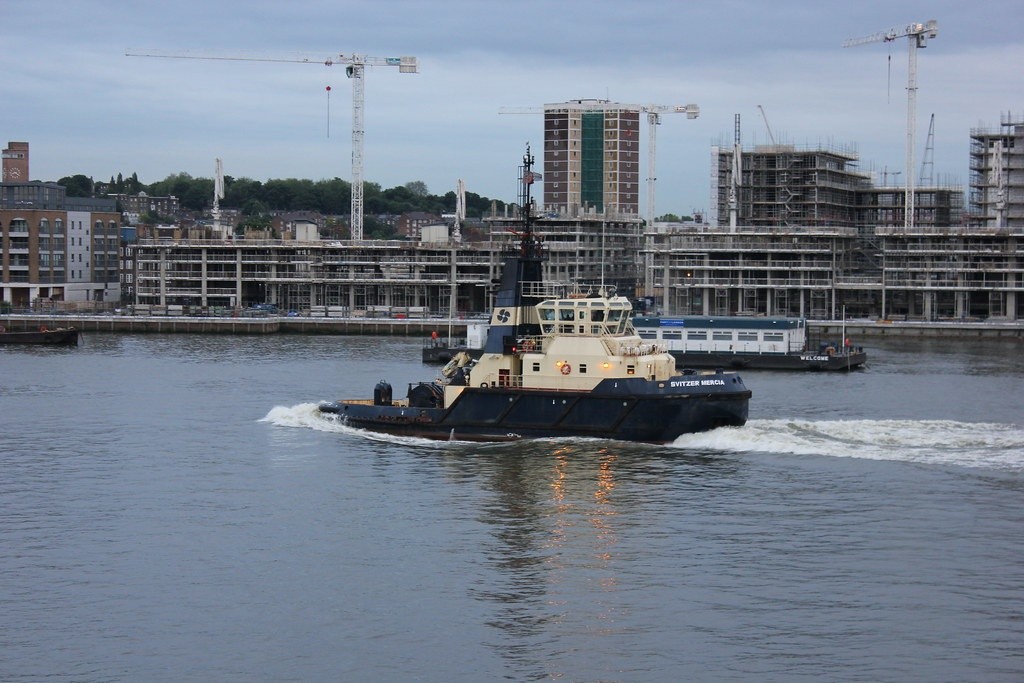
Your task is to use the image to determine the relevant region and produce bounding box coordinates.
[317,142,753,442]
[420,298,867,373]
[0,328,79,346]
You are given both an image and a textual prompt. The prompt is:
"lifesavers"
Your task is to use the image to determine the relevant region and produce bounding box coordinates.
[523,342,533,352]
[560,364,571,375]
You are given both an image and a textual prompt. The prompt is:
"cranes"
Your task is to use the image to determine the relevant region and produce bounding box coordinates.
[133,54,418,239]
[498,103,700,297]
[840,20,939,227]
